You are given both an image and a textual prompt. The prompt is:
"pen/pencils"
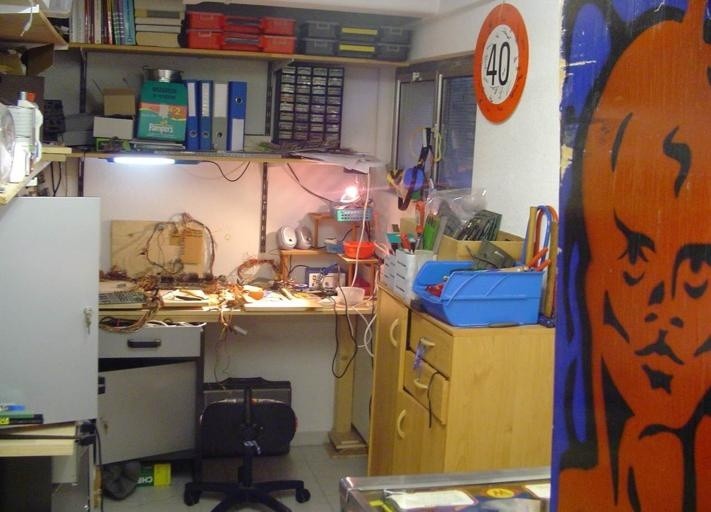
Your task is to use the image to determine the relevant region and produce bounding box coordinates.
[176,289,205,301]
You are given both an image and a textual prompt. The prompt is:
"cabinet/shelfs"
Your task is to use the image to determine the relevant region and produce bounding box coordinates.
[96,323,205,484]
[60,6,412,166]
[367,280,554,478]
[0,6,70,206]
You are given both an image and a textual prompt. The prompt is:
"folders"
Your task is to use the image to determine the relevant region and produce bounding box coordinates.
[183,80,248,151]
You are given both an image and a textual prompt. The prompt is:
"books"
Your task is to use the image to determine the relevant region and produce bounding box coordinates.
[72,1,185,48]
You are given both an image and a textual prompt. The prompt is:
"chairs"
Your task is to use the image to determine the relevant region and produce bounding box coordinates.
[184,377,310,512]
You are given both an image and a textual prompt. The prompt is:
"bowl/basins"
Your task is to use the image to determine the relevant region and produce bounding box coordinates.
[336,286,364,306]
[342,241,376,259]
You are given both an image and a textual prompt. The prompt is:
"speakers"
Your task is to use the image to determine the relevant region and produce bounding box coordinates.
[295,226,312,249]
[278,225,297,249]
